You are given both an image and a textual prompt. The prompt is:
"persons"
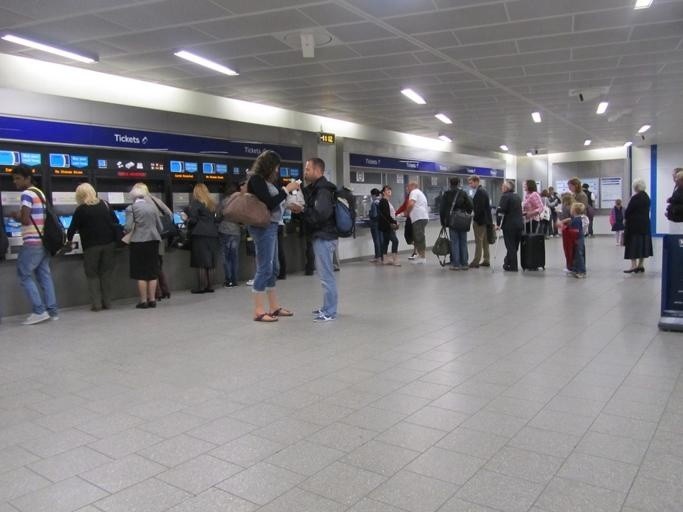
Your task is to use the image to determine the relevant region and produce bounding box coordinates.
[467,174,491,268]
[242,150,297,322]
[522,180,542,233]
[665,167,683,222]
[539,187,560,239]
[497,181,522,272]
[290,159,341,322]
[439,177,472,270]
[181,179,242,293]
[11,162,59,324]
[624,179,653,273]
[556,178,594,278]
[610,199,625,246]
[395,181,429,264]
[122,182,173,308]
[66,183,120,312]
[368,185,400,267]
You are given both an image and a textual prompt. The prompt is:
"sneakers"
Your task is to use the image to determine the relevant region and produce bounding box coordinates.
[564,268,586,278]
[312,309,323,315]
[450,262,489,270]
[21,310,60,324]
[409,252,425,264]
[502,263,517,271]
[368,254,401,266]
[312,314,336,322]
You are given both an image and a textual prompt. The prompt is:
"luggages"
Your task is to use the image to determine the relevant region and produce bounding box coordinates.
[520,213,544,271]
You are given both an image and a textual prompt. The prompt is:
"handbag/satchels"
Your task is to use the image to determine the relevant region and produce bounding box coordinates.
[451,210,472,231]
[404,217,414,243]
[113,222,127,251]
[433,225,449,254]
[391,223,399,229]
[487,224,497,244]
[223,192,270,227]
[161,214,192,248]
[539,205,550,221]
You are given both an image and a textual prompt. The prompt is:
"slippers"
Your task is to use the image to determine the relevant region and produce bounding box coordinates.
[272,308,292,316]
[254,314,277,322]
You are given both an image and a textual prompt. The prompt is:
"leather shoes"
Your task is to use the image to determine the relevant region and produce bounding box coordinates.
[625,267,644,273]
[135,301,158,309]
[191,288,214,294]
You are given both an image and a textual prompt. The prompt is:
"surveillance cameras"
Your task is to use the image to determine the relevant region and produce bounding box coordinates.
[534,149,539,154]
[641,133,648,140]
[578,88,601,103]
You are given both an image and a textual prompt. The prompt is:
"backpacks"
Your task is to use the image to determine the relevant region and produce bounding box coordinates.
[331,186,355,239]
[25,189,65,256]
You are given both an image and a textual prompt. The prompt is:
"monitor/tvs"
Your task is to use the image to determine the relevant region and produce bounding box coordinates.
[289,166,301,178]
[169,160,186,174]
[114,208,127,226]
[1,216,27,236]
[174,211,185,224]
[48,153,71,169]
[183,161,199,174]
[211,163,227,175]
[0,149,21,173]
[280,166,291,179]
[21,148,42,171]
[58,215,74,229]
[202,161,216,177]
[68,155,89,169]
